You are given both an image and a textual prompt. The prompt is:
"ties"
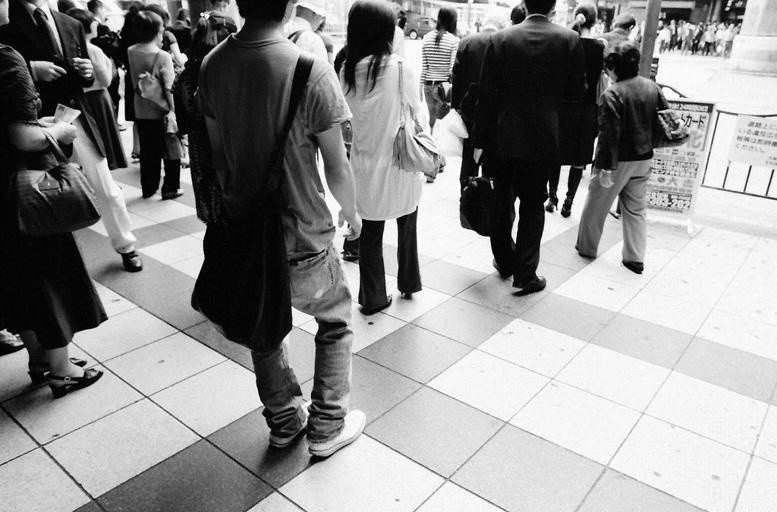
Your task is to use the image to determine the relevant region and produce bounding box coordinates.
[34,8,64,62]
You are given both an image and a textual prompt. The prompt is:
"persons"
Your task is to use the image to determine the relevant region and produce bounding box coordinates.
[1,1,741,356]
[0,0,109,399]
[192,0,368,461]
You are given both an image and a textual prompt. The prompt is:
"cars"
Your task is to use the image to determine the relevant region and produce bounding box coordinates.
[404,18,438,40]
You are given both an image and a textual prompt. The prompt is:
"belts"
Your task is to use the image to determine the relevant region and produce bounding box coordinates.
[423,80,444,86]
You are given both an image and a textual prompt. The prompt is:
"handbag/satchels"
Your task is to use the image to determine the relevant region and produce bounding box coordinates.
[459,162,515,237]
[190,184,291,354]
[135,69,170,112]
[652,107,690,152]
[15,127,102,239]
[392,123,447,178]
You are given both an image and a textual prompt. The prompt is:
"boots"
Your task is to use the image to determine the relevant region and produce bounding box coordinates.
[546,169,558,213]
[562,168,583,216]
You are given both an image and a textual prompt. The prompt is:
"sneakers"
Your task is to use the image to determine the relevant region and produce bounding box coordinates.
[269,400,312,448]
[307,409,366,460]
[164,189,183,201]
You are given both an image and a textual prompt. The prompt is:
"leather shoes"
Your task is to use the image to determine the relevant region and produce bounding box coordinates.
[513,278,548,290]
[27,357,103,397]
[121,251,142,271]
[623,261,643,274]
[360,296,391,315]
